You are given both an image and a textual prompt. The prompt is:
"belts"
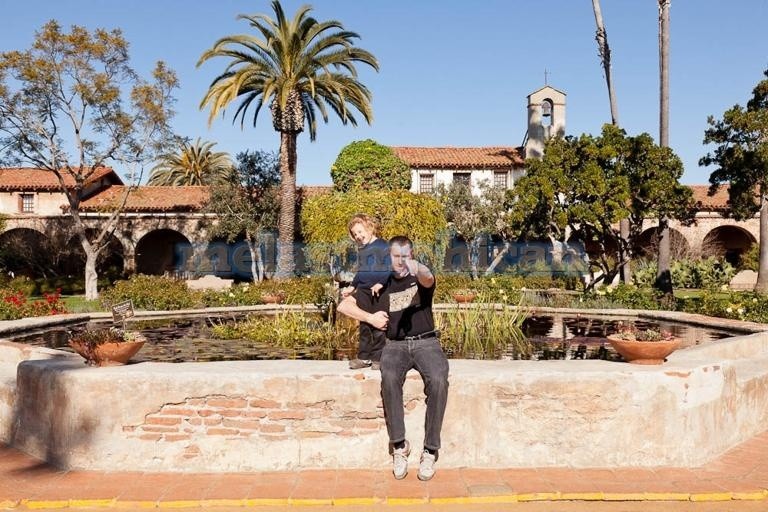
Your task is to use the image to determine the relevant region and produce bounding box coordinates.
[403,331,437,341]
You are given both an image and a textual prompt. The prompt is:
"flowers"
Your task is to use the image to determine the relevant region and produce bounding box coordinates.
[620,322,674,341]
[71,324,141,366]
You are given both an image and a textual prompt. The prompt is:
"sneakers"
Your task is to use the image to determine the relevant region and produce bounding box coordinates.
[417,451,437,481]
[349,358,372,369]
[393,439,412,480]
[371,361,381,370]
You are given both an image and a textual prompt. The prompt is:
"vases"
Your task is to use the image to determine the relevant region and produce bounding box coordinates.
[259,295,281,304]
[607,333,681,364]
[69,337,147,366]
[452,293,476,303]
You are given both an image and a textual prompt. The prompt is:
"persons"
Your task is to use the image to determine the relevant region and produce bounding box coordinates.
[336,234,451,482]
[343,216,394,370]
